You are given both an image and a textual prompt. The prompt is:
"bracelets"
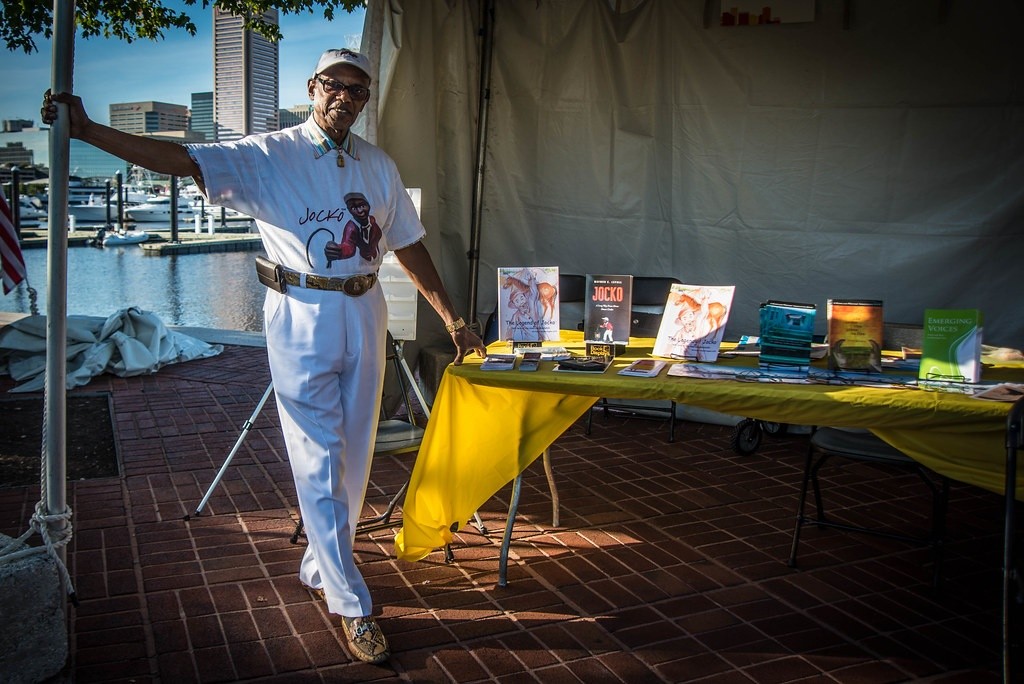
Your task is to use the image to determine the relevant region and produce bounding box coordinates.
[444,317,465,332]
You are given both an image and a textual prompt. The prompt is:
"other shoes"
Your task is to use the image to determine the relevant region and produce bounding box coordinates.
[341,615,391,664]
[302,581,328,605]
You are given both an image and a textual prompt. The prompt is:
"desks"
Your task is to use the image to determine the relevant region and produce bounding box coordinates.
[392,329,1024,589]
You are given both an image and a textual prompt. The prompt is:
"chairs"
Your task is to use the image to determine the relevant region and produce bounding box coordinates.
[485,275,683,441]
[291,328,454,563]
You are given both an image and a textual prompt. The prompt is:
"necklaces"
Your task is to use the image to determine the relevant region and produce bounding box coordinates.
[335,146,346,168]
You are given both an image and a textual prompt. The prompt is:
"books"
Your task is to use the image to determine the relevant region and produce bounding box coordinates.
[478,267,984,384]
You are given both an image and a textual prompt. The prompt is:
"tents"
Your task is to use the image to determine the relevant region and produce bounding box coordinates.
[351,0,1024,436]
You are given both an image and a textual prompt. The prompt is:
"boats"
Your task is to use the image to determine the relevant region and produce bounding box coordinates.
[0,174,253,232]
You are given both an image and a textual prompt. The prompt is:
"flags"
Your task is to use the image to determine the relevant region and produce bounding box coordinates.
[0,183,27,295]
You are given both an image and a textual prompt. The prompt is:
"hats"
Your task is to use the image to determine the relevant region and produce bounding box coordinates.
[312,48,371,88]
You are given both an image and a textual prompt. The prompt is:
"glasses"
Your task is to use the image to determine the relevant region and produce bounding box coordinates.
[317,75,370,101]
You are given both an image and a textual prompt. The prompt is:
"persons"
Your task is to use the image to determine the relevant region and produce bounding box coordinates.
[40,49,486,665]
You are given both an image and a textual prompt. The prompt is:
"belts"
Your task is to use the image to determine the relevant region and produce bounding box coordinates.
[283,268,380,297]
